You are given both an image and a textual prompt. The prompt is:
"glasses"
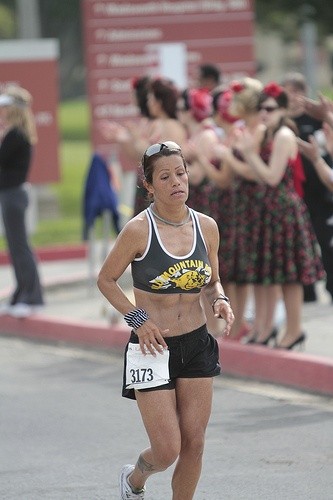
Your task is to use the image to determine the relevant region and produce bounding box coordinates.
[255,105,277,112]
[143,141,182,175]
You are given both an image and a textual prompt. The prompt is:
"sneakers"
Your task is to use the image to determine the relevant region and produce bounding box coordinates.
[119,464,146,500]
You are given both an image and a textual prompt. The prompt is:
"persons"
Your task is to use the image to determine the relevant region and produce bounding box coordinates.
[98,140,236,500]
[0,86,43,318]
[133,63,333,351]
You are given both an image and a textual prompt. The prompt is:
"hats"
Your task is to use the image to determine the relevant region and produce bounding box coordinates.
[0,93,27,107]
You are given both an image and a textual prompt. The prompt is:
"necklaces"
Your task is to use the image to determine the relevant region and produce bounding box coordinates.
[151,205,190,227]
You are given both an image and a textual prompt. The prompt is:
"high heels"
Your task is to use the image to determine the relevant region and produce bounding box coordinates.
[272,330,305,352]
[228,323,251,343]
[253,328,276,346]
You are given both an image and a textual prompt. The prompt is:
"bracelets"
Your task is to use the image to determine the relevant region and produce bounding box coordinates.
[212,294,230,319]
[124,309,148,328]
